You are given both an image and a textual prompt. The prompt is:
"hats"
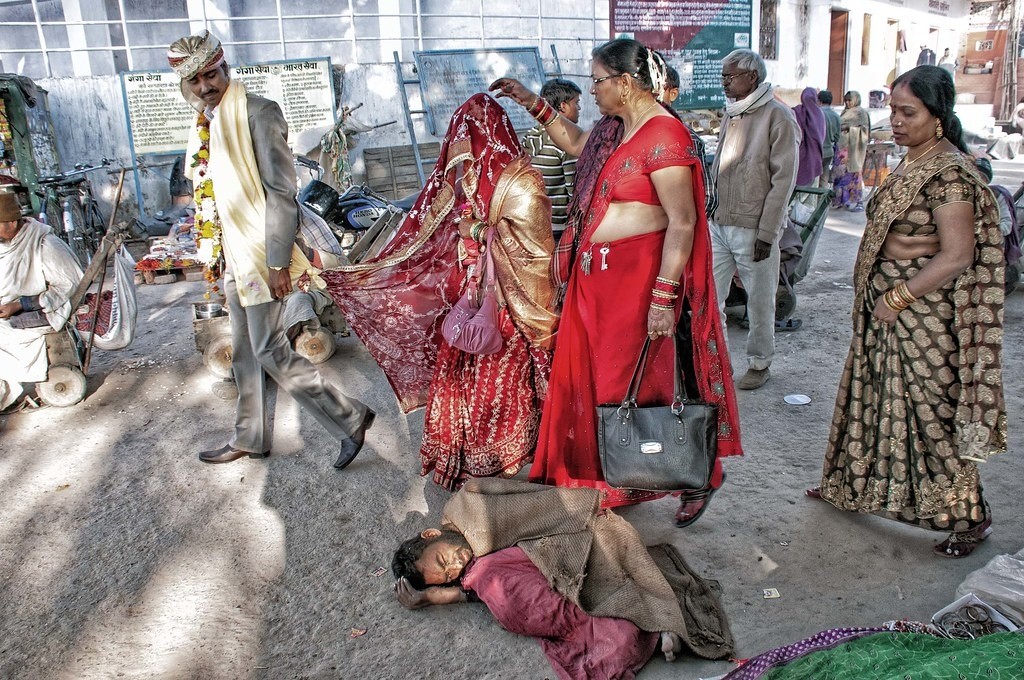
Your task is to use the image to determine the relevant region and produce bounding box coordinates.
[974,158,992,184]
[167,29,225,81]
[0,191,23,222]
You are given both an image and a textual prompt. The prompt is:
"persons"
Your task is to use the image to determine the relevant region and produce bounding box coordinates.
[660,65,719,221]
[520,80,583,245]
[0,192,85,415]
[936,48,962,84]
[398,92,569,491]
[792,87,871,213]
[706,48,799,391]
[166,30,377,471]
[391,478,683,680]
[973,157,1023,266]
[488,40,744,529]
[805,65,1008,560]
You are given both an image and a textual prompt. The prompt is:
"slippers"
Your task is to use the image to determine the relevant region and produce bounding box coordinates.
[932,526,993,558]
[805,486,824,500]
[674,471,726,528]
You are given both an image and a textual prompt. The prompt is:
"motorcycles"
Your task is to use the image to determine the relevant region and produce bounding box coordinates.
[292,156,423,250]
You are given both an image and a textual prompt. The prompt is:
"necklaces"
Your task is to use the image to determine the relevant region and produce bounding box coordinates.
[616,102,659,151]
[903,137,946,171]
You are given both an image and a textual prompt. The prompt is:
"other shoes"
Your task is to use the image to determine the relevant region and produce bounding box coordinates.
[738,366,772,390]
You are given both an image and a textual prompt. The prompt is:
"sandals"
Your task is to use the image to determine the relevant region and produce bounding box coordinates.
[740,312,802,331]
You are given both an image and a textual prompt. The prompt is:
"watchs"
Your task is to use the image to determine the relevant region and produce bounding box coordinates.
[269,267,288,271]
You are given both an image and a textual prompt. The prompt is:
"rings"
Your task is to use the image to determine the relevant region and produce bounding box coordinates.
[647,330,655,335]
[883,321,888,325]
[871,315,878,321]
[662,330,667,333]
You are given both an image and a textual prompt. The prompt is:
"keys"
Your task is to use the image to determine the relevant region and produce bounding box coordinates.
[580,252,592,276]
[600,248,609,271]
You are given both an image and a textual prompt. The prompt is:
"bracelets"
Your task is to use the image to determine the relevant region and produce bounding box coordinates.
[469,221,489,244]
[650,277,680,311]
[882,282,918,313]
[526,93,560,127]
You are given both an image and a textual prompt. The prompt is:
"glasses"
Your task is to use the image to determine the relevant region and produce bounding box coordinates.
[593,73,639,84]
[720,71,749,83]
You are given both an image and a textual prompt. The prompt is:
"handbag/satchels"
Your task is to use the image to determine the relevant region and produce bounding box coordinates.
[595,401,720,493]
[441,227,502,355]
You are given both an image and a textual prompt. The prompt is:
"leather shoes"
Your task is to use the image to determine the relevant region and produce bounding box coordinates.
[199,444,271,464]
[333,406,376,470]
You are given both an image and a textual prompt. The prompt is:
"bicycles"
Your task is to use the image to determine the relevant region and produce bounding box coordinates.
[38,158,113,272]
